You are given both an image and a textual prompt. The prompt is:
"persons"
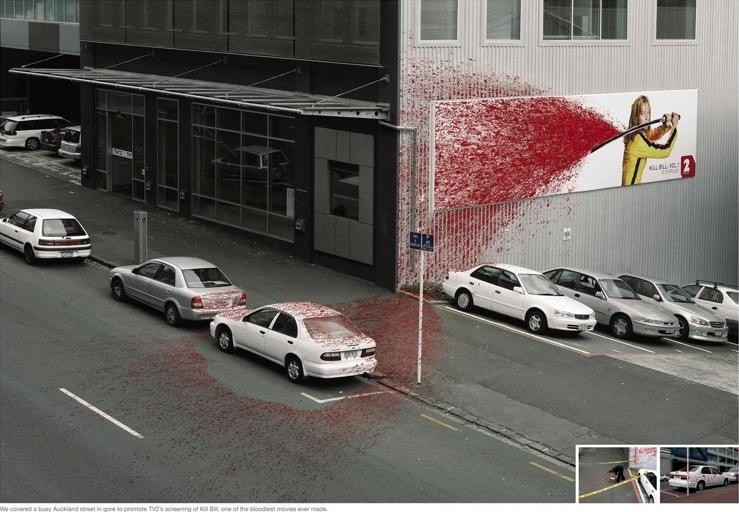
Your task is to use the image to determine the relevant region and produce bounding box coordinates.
[622,95,680,185]
[606,465,625,483]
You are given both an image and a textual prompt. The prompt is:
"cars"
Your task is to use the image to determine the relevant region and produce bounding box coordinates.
[0,208,92,266]
[442,264,739,346]
[210,302,378,387]
[723,467,739,483]
[661,473,670,482]
[668,464,729,490]
[211,146,292,186]
[638,467,657,502]
[110,255,246,326]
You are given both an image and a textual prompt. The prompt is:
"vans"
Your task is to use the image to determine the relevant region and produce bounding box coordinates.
[0,113,82,161]
